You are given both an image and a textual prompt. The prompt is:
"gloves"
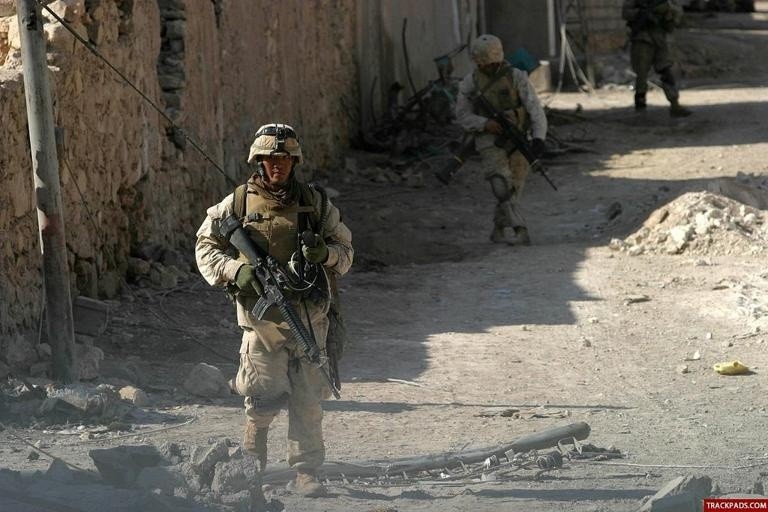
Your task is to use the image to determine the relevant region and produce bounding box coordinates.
[236,265,264,296]
[530,139,544,162]
[302,234,329,264]
[485,120,505,136]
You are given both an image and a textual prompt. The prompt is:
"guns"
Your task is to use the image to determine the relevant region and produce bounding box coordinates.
[218,215,341,399]
[472,95,558,193]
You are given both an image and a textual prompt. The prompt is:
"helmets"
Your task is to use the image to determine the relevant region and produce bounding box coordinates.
[247,122,304,166]
[470,33,505,66]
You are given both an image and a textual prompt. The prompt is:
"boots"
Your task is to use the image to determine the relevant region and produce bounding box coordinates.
[507,227,531,245]
[242,419,268,474]
[490,226,509,244]
[293,469,327,496]
[669,100,692,118]
[636,94,646,109]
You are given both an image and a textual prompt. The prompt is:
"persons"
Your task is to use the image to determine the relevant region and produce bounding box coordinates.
[457,34,551,246]
[620,0,694,115]
[194,122,353,497]
